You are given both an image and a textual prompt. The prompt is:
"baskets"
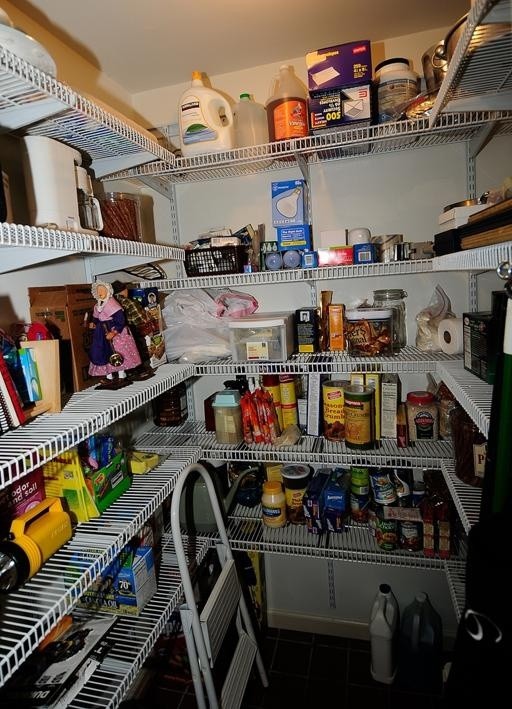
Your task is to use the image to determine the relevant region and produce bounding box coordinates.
[184,237,249,277]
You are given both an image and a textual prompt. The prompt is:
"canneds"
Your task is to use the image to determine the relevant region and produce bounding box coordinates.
[351,459,425,551]
[263,374,301,430]
[322,380,376,449]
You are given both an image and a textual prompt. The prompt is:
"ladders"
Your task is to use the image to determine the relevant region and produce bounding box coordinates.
[167,462,271,707]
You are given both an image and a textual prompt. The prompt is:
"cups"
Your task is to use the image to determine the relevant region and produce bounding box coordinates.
[392,240,434,261]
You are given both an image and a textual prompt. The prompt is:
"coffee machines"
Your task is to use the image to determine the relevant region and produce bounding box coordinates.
[21,130,106,239]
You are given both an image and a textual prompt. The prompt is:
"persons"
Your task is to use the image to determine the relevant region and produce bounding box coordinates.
[86,281,143,386]
[149,293,156,303]
[111,280,155,375]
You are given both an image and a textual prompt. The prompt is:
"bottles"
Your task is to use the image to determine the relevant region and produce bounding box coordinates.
[372,286,408,352]
[260,463,311,530]
[407,384,457,442]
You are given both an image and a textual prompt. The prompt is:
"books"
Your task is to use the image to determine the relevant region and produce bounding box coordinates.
[0,355,25,435]
[458,198,512,251]
